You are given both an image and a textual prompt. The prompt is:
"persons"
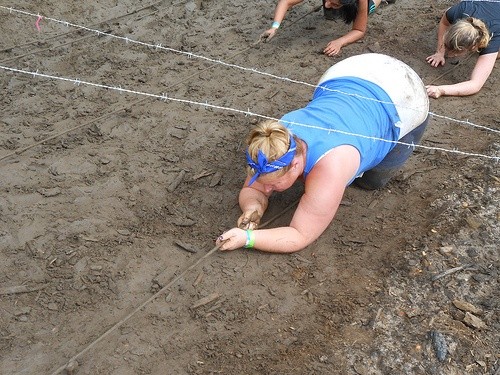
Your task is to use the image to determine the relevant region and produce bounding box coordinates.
[216,53,430,254]
[425,0,500,99]
[259,0,396,56]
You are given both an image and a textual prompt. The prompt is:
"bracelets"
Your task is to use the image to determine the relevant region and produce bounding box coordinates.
[272,21,281,28]
[244,229,255,249]
